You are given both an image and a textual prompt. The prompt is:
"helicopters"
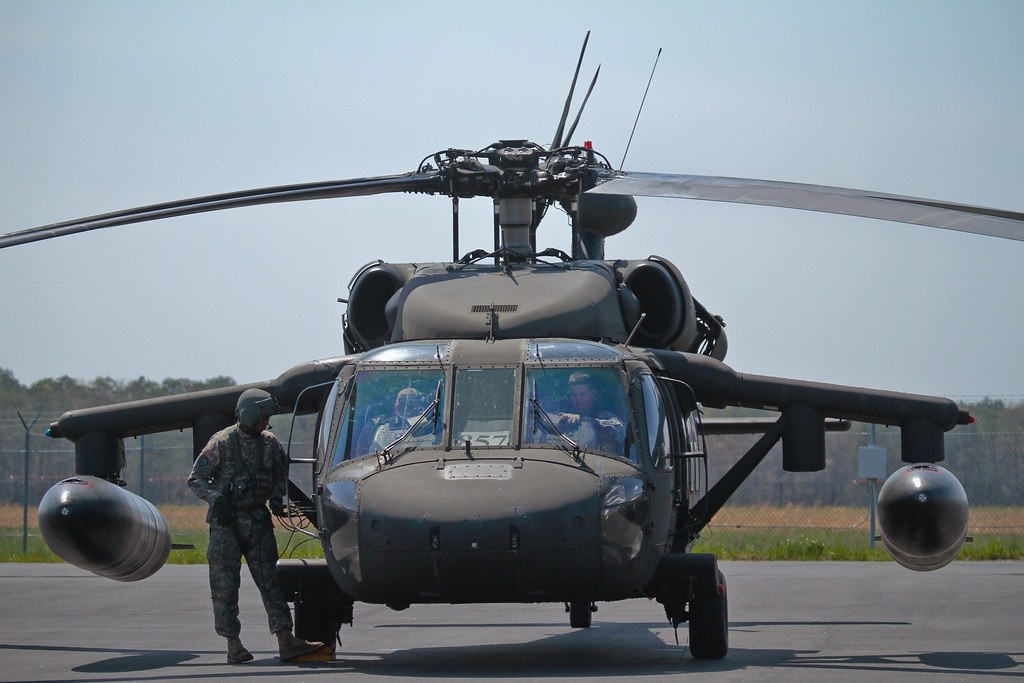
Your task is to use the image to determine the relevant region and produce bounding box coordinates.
[0,31,1024,660]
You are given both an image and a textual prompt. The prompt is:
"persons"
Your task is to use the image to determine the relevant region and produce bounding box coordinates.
[187,389,323,665]
[560,372,626,456]
[354,388,424,457]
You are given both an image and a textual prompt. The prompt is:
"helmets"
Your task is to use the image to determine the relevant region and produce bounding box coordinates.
[236,388,280,427]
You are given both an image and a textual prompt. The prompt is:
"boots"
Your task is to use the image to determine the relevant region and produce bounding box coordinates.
[227,636,253,664]
[276,628,325,661]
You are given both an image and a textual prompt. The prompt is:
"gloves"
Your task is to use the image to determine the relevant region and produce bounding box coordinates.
[213,501,232,528]
